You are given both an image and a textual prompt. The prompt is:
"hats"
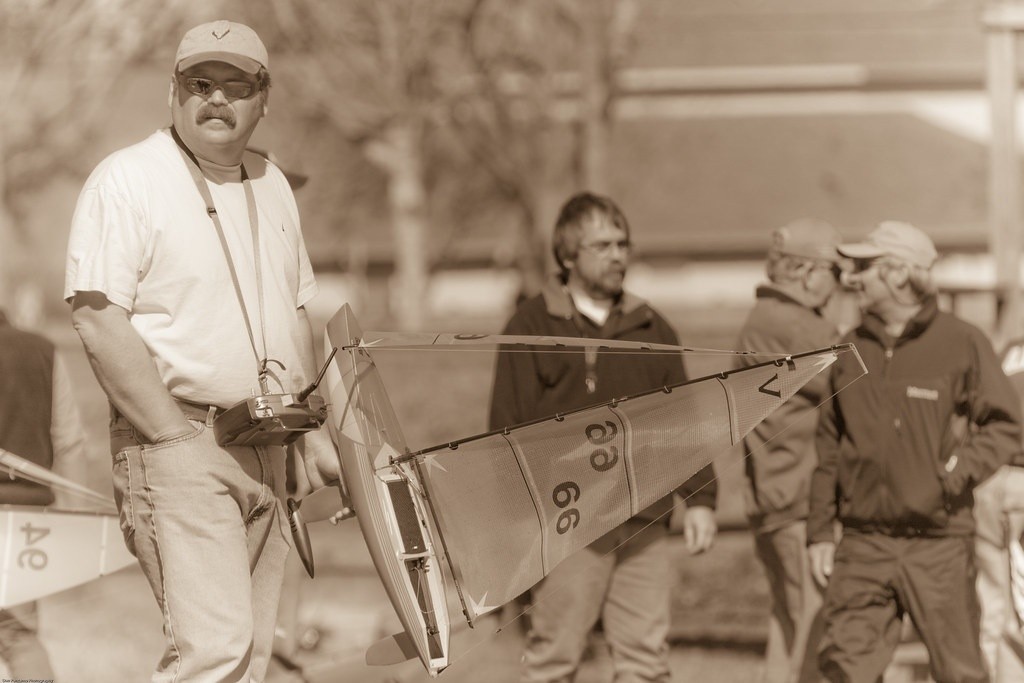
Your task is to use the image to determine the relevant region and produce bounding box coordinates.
[837,221,938,274]
[175,19,268,75]
[774,217,845,263]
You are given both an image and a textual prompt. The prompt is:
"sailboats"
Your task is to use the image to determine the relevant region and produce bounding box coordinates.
[320,302,868,682]
[2,450,146,617]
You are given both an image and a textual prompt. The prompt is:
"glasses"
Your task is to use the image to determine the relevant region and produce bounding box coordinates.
[578,242,635,256]
[175,71,262,100]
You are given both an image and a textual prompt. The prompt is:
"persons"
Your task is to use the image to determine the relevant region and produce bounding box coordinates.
[970,336,1024,683]
[730,219,847,683]
[486,190,719,683]
[63,19,342,683]
[805,220,1024,683]
